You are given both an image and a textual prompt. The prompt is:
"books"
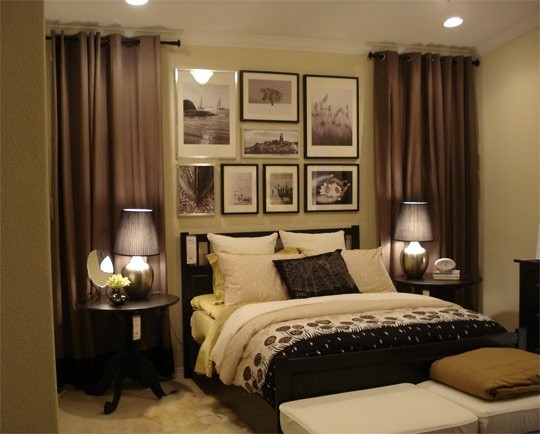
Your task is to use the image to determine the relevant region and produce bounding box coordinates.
[432,270,460,280]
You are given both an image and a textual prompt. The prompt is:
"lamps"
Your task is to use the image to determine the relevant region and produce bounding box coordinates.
[393,201,434,279]
[114,209,161,301]
[97,250,113,274]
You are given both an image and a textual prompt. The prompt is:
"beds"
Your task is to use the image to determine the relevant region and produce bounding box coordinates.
[180,225,527,434]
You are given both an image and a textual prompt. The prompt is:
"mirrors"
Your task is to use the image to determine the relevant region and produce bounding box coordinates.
[87,249,113,302]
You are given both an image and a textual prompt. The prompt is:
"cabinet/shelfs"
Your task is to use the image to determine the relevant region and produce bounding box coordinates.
[513,259,540,354]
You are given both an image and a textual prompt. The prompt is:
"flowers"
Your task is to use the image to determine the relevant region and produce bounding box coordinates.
[106,274,131,288]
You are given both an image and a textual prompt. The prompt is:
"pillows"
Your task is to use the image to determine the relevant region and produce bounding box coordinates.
[272,249,359,299]
[299,247,394,293]
[217,252,306,305]
[208,247,298,306]
[279,229,346,248]
[208,233,278,252]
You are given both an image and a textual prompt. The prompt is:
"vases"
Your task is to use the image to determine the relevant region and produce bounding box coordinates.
[106,288,128,304]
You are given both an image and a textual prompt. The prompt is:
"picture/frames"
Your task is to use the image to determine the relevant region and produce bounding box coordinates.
[302,74,359,160]
[176,164,215,217]
[303,163,359,212]
[240,128,300,158]
[221,163,259,215]
[174,68,236,160]
[262,163,299,214]
[241,70,298,124]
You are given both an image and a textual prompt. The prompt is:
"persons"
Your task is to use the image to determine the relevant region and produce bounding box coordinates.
[312,173,350,204]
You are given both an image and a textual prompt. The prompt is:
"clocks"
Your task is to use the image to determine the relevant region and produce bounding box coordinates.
[434,257,456,271]
